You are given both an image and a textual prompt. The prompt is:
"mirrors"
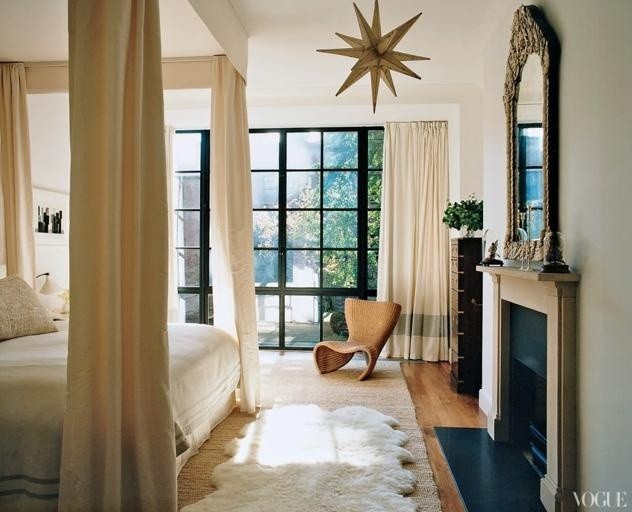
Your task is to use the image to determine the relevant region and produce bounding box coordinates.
[503,4,562,264]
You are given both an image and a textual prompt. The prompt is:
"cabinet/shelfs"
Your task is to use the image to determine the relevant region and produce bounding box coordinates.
[449,238,482,394]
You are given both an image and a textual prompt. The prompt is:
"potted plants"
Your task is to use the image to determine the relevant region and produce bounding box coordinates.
[442,197,483,238]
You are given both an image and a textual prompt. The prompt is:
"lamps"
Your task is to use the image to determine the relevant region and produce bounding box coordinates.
[34,272,57,296]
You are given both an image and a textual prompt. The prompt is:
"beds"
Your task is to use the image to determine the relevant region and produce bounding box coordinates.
[1,323,239,512]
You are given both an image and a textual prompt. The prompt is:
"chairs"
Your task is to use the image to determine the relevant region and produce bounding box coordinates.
[314,297,400,380]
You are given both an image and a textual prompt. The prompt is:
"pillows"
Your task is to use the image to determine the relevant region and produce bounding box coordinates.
[1,276,58,342]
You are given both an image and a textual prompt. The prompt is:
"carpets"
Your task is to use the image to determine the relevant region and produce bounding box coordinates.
[180,361,547,512]
